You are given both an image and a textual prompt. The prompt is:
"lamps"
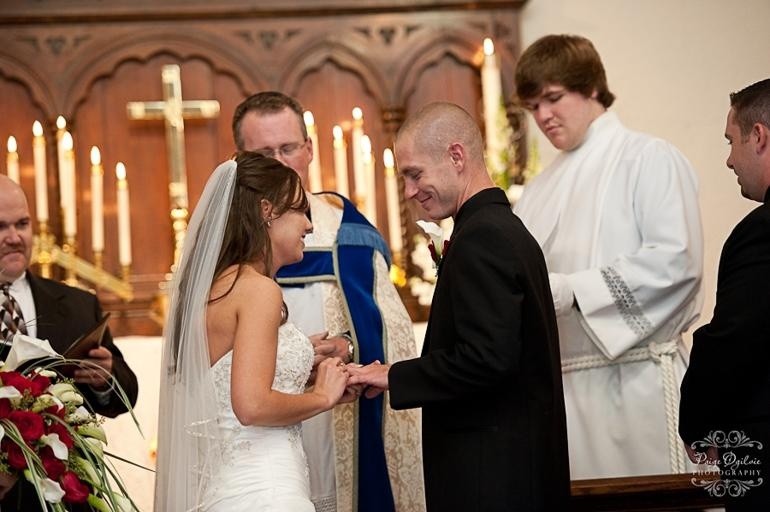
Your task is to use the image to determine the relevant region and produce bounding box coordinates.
[4,115,134,265]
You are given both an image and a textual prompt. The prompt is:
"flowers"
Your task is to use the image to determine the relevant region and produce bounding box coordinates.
[0,265,163,512]
[414,217,453,280]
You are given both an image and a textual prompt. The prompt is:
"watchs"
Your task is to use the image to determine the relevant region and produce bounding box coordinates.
[343,335,355,362]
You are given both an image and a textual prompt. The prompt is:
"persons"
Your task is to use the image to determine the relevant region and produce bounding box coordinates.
[232,90,395,512]
[347,103,570,511]
[677,81,770,512]
[154,149,366,512]
[510,34,703,482]
[0,173,138,511]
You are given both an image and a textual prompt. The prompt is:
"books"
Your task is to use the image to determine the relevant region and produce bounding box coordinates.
[1,313,111,386]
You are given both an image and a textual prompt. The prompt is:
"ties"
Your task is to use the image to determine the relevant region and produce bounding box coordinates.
[0,280,30,343]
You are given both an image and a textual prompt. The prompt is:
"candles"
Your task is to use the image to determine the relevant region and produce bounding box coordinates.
[480,36,506,175]
[302,104,406,253]
